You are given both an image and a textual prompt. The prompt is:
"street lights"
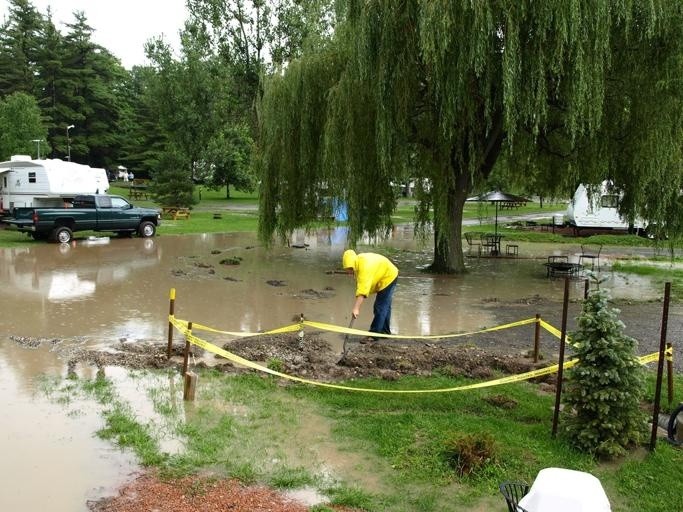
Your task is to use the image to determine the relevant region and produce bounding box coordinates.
[66,125,75,161]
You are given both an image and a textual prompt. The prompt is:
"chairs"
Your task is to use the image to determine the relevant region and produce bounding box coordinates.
[500,482,532,512]
[578,242,603,272]
[464,232,481,256]
[547,256,570,279]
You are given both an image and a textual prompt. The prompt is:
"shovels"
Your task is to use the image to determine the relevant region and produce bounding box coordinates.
[334,315,356,365]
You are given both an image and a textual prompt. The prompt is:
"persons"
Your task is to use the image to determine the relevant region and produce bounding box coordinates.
[122,171,135,186]
[341,248,399,345]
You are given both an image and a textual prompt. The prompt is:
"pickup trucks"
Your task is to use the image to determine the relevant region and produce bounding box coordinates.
[14,235,162,285]
[0,194,161,243]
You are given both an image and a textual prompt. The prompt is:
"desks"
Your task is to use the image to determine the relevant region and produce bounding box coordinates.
[161,207,191,220]
[480,235,505,256]
[542,263,580,281]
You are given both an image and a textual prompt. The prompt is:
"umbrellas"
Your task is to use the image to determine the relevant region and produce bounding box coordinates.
[465,189,533,251]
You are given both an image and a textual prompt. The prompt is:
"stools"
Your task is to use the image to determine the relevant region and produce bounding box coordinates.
[506,244,518,256]
